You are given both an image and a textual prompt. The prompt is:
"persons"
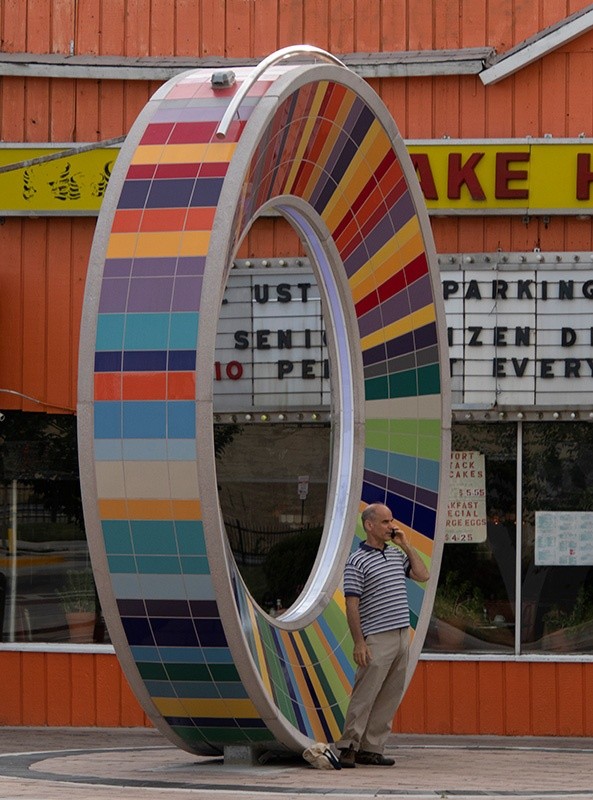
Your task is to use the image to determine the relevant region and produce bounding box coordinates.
[336,504,430,768]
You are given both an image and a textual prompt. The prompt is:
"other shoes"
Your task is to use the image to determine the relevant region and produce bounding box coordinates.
[339,749,356,768]
[355,750,396,766]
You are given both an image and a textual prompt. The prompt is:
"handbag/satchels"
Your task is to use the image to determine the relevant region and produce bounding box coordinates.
[302,743,342,771]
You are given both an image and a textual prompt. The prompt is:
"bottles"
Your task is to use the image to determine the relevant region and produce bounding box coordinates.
[482,608,488,626]
[493,615,506,626]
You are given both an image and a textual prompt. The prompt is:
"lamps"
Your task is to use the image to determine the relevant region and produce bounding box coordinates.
[210,70,237,89]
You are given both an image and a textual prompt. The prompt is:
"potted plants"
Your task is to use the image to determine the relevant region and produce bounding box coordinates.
[56,550,98,643]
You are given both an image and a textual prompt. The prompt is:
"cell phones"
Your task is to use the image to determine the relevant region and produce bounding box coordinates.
[391,528,396,539]
